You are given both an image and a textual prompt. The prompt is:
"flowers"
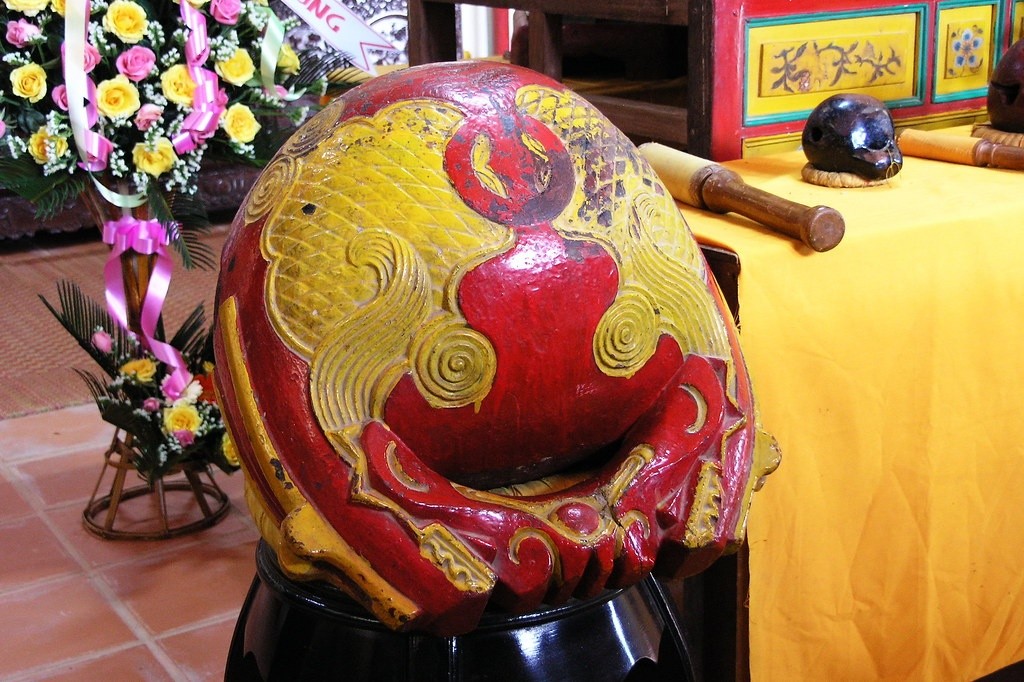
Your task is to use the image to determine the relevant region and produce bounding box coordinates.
[1,0,375,495]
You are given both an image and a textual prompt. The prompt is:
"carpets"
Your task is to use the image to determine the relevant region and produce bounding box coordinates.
[0,222,232,421]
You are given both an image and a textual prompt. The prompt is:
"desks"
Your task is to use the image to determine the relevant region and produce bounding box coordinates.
[676,124,1024,681]
